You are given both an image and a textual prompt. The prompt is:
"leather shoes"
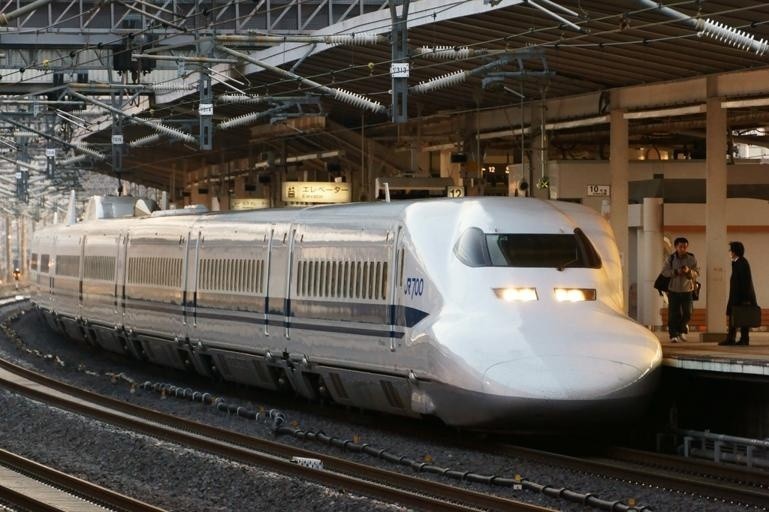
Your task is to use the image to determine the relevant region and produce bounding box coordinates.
[719,339,749,345]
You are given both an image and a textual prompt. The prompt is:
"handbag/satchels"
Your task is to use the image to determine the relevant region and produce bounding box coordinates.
[655,256,674,291]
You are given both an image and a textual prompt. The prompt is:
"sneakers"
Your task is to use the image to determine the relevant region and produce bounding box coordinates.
[670,333,687,343]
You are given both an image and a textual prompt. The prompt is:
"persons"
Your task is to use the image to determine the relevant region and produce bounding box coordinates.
[717,240,757,345]
[662,238,700,345]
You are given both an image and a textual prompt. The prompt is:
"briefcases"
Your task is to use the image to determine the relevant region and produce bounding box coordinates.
[730,304,761,327]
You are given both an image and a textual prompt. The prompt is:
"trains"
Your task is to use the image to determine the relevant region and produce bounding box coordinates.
[27,196,663,429]
